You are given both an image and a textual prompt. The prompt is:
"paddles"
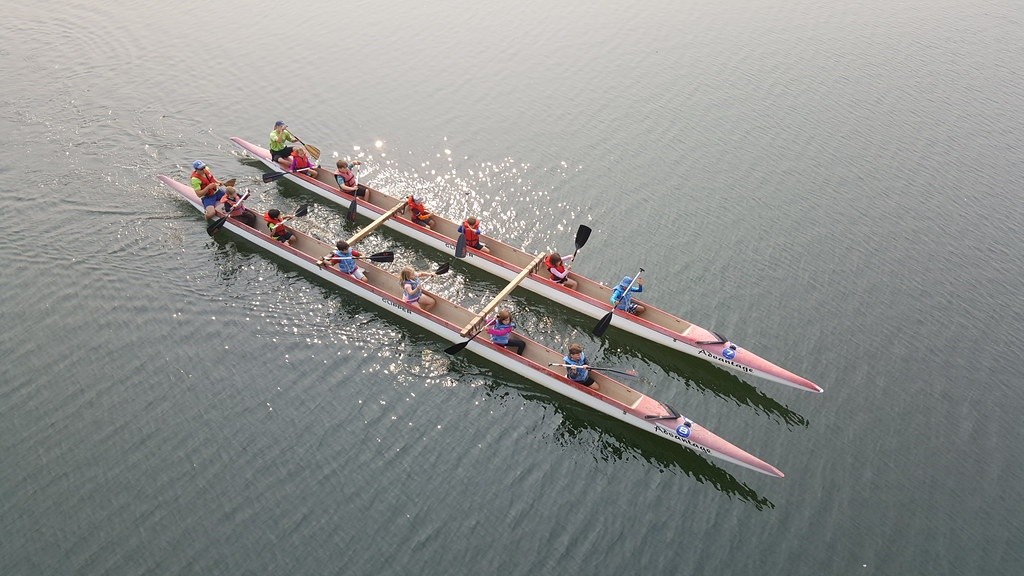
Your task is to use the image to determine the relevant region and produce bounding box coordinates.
[285,129,321,160]
[270,204,308,232]
[443,315,500,355]
[421,262,450,285]
[207,189,249,237]
[455,193,470,258]
[570,224,592,265]
[592,267,645,337]
[322,251,394,262]
[215,179,236,187]
[548,362,639,378]
[406,197,437,217]
[346,162,360,223]
[263,165,321,183]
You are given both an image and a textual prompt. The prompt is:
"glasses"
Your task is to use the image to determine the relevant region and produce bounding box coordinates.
[199,167,206,171]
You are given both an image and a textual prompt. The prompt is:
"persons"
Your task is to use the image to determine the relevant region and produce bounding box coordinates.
[270,120,298,166]
[290,147,318,180]
[400,267,436,312]
[264,209,297,244]
[320,240,369,283]
[457,216,490,253]
[334,160,370,203]
[191,159,226,220]
[220,186,256,226]
[409,194,435,229]
[545,252,577,291]
[563,343,599,390]
[609,275,645,316]
[483,310,525,356]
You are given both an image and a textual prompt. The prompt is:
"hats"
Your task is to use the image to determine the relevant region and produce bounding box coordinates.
[620,276,633,289]
[413,193,423,202]
[276,120,285,127]
[193,161,206,169]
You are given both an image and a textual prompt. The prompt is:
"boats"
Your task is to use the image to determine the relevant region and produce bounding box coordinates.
[228,136,827,396]
[156,174,785,479]
